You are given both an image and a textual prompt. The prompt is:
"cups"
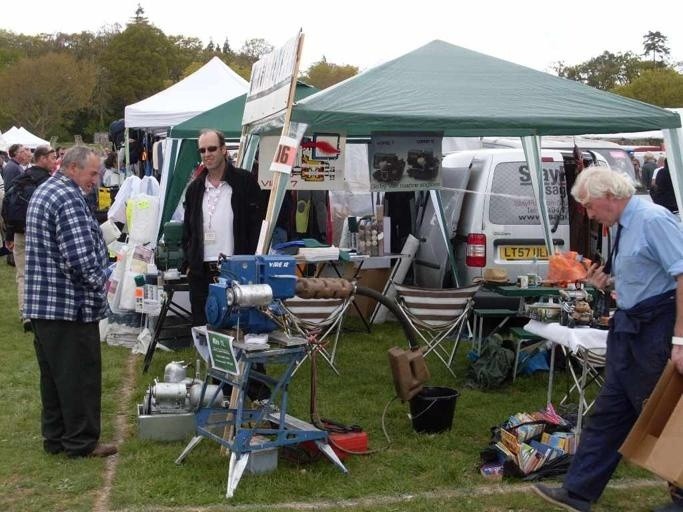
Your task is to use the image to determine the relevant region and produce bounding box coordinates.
[517,273,542,289]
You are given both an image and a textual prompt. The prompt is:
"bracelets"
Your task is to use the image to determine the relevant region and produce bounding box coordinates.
[671,335,683,346]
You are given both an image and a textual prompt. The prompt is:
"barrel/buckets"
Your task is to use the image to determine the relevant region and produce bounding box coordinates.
[407,387,461,435]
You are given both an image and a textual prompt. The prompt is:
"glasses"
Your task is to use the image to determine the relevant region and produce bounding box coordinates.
[196,146,222,153]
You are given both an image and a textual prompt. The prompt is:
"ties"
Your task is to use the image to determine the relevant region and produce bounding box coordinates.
[601,223,625,275]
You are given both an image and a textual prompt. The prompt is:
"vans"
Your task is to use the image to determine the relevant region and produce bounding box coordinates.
[411,147,614,318]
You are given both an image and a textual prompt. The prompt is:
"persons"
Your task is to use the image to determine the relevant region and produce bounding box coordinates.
[22,145,119,459]
[0,144,128,267]
[529,166,683,511]
[180,131,270,404]
[1,144,58,333]
[630,152,679,213]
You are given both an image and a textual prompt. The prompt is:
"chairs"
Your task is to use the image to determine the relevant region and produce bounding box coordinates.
[545,343,604,435]
[389,277,482,378]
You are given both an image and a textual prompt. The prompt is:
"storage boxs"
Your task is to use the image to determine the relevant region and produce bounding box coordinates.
[343,258,392,318]
[618,358,683,492]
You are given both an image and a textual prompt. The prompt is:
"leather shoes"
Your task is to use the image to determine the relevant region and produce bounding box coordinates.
[531,483,592,511]
[86,443,120,455]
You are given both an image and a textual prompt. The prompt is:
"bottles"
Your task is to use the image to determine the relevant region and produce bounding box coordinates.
[134,271,163,309]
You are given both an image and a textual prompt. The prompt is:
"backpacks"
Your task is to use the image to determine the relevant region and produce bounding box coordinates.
[0,169,51,234]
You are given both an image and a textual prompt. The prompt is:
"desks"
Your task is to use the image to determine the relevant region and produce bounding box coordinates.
[482,285,595,315]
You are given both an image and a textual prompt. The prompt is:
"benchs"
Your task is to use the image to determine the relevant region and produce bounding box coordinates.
[471,307,545,385]
